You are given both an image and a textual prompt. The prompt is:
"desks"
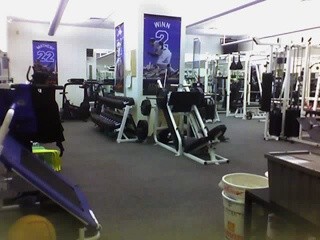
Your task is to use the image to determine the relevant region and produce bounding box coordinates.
[242,188,320,240]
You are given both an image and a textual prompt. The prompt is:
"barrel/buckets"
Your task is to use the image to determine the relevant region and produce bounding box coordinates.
[218,173,271,240]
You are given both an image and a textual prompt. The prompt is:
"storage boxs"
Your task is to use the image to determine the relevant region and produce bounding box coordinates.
[264,150,320,227]
